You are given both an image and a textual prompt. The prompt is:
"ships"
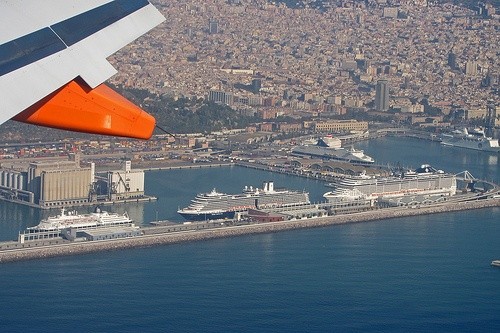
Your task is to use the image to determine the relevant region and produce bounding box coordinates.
[320,166,456,204]
[438,126,500,153]
[27,206,134,239]
[291,135,375,167]
[176,180,311,220]
